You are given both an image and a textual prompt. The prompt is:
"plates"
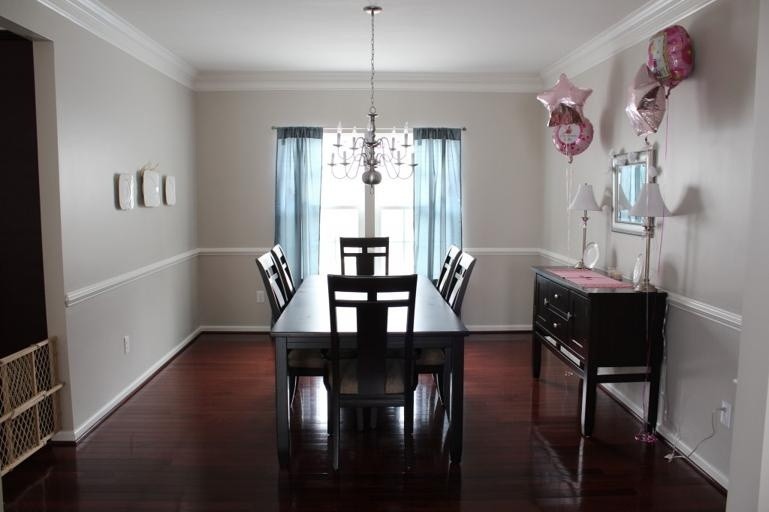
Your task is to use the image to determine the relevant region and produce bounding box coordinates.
[582,242,600,270]
[632,253,644,285]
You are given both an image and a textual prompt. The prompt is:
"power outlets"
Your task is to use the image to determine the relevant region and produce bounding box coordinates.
[719,400,732,429]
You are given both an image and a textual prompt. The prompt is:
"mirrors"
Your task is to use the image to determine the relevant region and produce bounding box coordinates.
[611,151,656,237]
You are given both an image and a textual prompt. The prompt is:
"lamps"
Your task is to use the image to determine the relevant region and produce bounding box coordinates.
[328,0,417,194]
[568,182,603,268]
[627,182,672,293]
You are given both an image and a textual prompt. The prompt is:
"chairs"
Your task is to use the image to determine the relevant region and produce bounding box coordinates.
[325,273,420,468]
[256,235,477,408]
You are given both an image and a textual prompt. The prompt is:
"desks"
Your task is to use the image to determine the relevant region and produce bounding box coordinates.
[270,272,470,466]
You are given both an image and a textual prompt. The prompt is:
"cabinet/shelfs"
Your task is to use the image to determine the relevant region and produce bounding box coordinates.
[531,264,668,437]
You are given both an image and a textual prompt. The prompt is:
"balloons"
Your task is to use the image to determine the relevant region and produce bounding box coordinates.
[625,64,667,140]
[646,25,693,91]
[535,73,593,127]
[552,114,593,158]
